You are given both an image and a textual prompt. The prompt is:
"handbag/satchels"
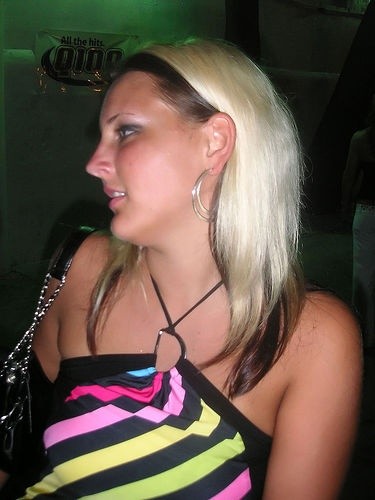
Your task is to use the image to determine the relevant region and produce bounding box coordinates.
[0,225,94,480]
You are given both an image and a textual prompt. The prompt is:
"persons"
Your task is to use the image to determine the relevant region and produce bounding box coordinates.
[0,36,364,500]
[342,126,375,350]
